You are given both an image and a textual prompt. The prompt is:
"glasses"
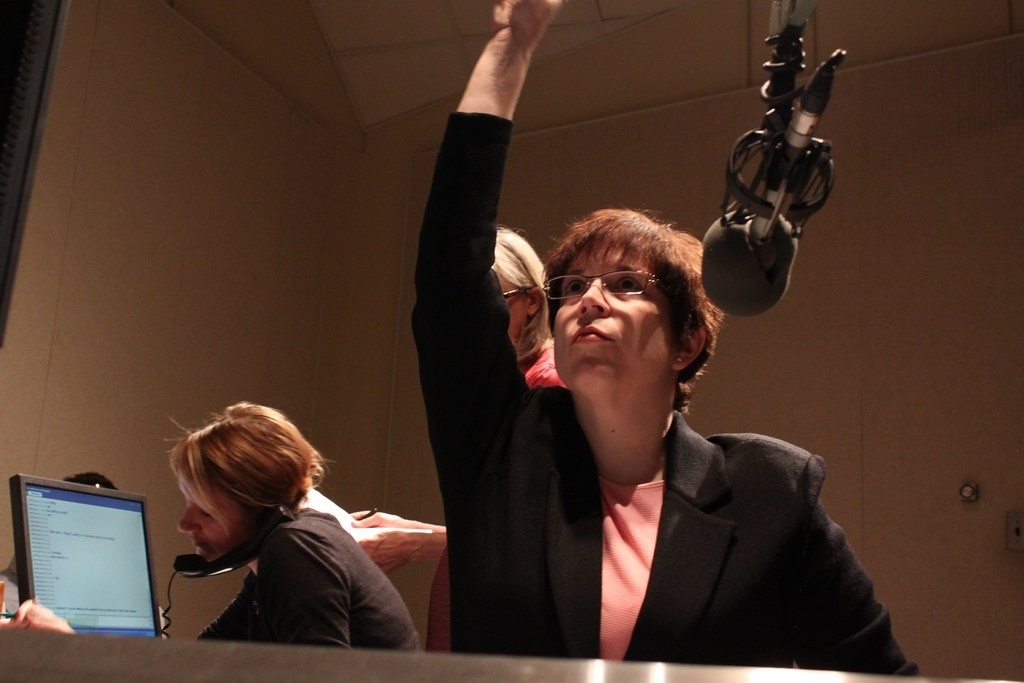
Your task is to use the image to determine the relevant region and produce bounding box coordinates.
[543,271,683,308]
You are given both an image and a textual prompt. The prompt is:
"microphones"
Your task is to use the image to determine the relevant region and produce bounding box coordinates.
[701,50,845,318]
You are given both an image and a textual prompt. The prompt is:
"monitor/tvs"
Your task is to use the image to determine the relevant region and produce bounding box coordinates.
[8,472,162,638]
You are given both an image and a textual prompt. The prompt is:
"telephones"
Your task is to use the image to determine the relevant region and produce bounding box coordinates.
[172,505,296,578]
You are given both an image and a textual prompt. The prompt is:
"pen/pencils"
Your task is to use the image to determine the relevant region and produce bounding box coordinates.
[358,508,378,520]
[0,611,15,618]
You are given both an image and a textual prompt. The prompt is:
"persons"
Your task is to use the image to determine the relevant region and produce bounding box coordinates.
[348,226,569,653]
[0,403,424,652]
[0,472,118,615]
[411,1,920,677]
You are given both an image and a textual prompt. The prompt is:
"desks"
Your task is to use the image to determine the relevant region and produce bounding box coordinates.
[0,625,879,683]
